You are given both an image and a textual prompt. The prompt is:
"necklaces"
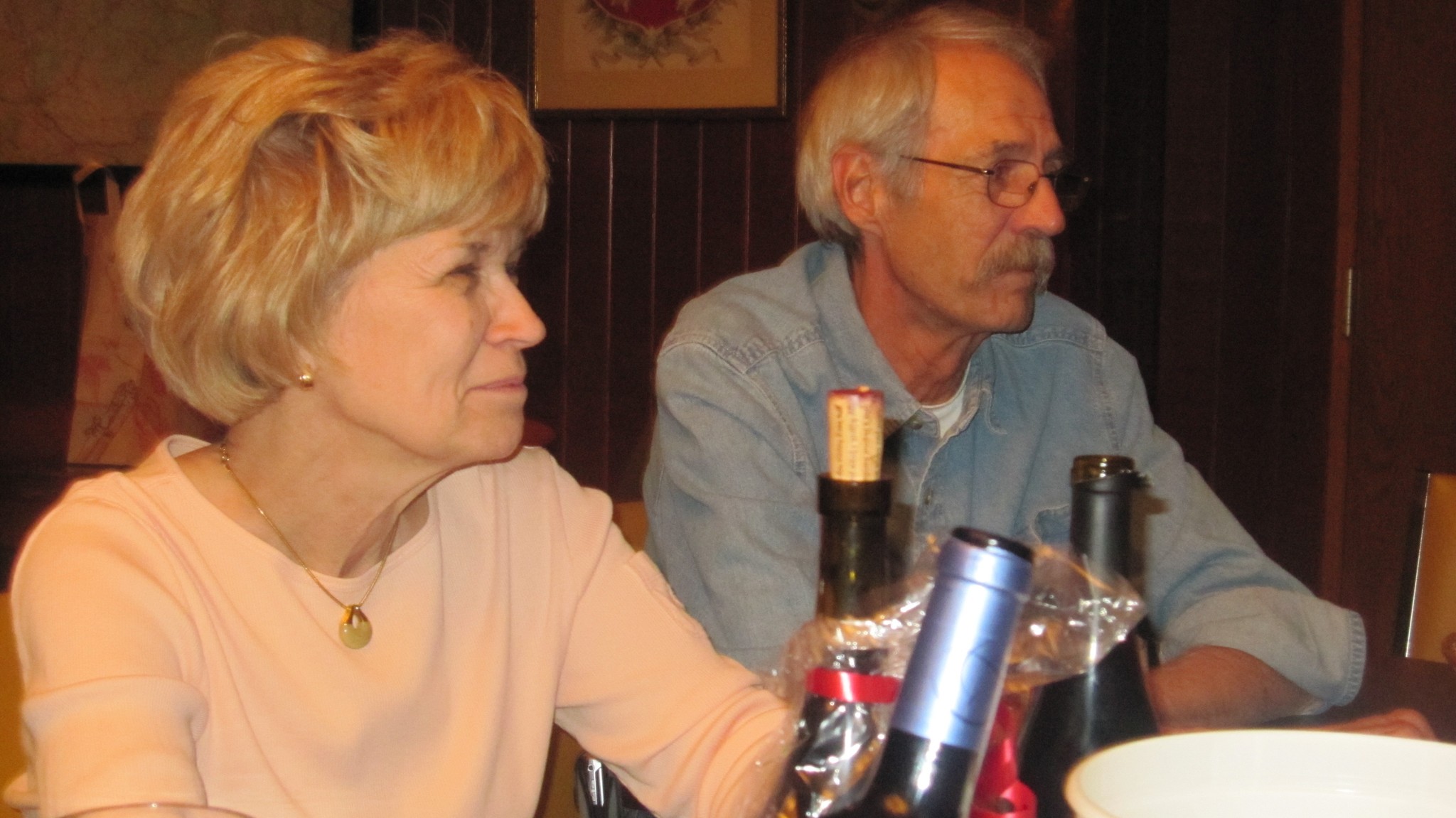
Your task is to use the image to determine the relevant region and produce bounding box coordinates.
[213,434,390,648]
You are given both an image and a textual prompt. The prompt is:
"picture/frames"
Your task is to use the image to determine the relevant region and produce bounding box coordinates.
[525,0,791,120]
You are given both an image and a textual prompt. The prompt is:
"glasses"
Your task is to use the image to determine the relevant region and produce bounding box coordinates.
[865,147,1061,209]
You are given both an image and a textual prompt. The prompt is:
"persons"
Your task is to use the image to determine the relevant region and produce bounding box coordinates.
[5,34,812,817]
[642,12,1442,747]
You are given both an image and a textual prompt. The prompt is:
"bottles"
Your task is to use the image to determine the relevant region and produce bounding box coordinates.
[767,392,904,817]
[850,523,1036,818]
[1018,454,1164,817]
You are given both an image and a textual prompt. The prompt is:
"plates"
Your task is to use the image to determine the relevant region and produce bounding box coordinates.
[1063,728,1454,818]
